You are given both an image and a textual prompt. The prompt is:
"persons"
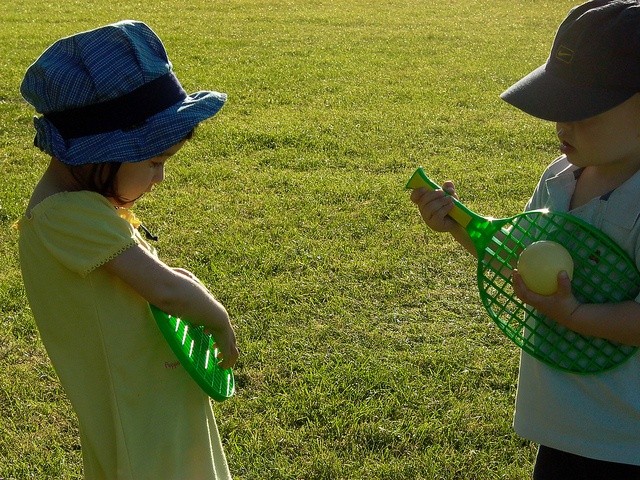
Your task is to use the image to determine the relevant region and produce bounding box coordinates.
[14,19,241,479]
[407,0,640,479]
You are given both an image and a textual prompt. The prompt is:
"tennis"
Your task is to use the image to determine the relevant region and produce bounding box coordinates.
[519,242,574,295]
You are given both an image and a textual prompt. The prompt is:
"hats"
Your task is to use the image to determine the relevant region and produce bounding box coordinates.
[498,0,639,122]
[21,22,224,166]
